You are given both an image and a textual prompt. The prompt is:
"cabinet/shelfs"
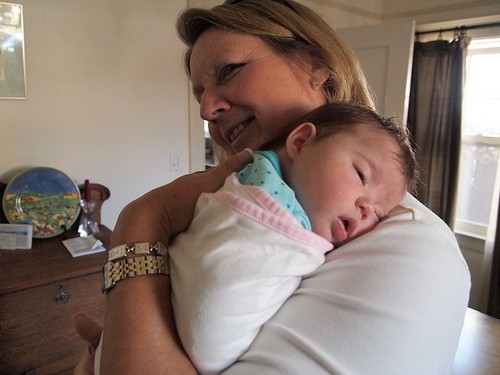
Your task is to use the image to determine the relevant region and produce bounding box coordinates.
[0,262,114,375]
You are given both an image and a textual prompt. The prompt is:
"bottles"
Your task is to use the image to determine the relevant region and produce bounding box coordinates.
[78,199,99,238]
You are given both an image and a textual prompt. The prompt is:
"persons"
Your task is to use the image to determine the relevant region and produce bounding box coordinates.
[66,0,473,375]
[92,101,420,374]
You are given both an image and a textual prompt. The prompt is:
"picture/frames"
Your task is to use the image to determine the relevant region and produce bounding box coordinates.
[0,2,27,100]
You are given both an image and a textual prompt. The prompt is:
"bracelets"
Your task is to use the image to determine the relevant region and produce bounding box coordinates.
[105,241,169,262]
[101,255,170,295]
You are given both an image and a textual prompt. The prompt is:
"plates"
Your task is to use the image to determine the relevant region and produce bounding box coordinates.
[2,167,82,237]
